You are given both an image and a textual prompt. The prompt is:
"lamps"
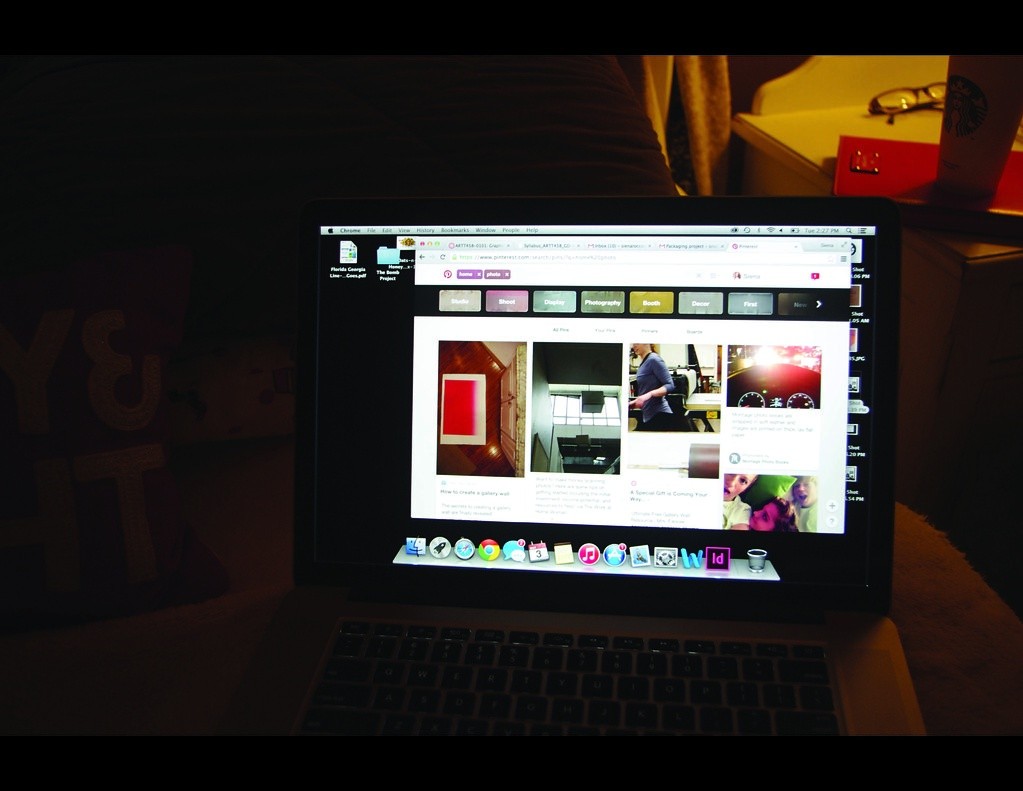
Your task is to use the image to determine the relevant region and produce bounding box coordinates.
[580,385,605,413]
[576,425,591,448]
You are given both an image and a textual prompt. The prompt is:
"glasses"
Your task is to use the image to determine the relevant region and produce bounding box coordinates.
[868,82,948,124]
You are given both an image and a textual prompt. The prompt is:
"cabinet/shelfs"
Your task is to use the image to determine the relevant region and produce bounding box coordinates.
[500,355,517,471]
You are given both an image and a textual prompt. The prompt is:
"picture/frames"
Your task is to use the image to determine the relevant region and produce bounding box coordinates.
[531,432,549,472]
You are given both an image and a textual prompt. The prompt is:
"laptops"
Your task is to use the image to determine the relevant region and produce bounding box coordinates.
[213,197,926,735]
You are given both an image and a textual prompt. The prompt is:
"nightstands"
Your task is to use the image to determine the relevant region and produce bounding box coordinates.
[726,112,1023,602]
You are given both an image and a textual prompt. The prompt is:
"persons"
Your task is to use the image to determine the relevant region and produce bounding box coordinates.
[723,473,816,531]
[629,343,674,431]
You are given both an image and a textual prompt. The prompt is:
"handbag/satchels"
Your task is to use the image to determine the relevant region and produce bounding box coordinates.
[834,137,1023,211]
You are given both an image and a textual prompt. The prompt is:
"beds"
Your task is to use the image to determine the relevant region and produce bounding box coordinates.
[0,52,1022,736]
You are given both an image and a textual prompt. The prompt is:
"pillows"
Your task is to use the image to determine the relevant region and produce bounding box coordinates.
[740,475,798,511]
[1,242,227,635]
